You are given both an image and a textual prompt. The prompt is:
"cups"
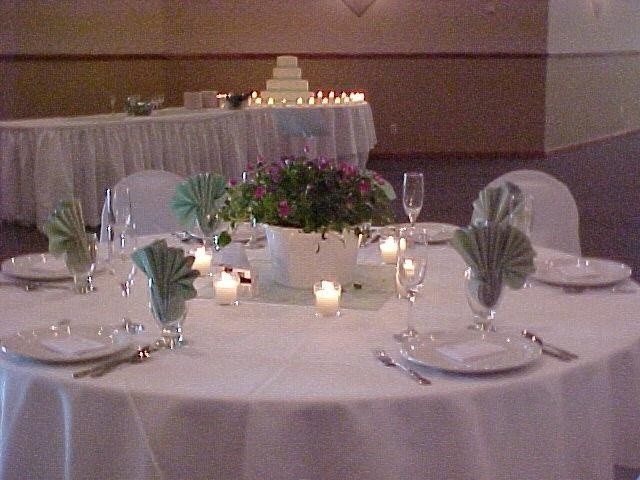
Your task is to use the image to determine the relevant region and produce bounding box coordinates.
[183,91,219,112]
[378,236,400,264]
[213,272,241,305]
[314,281,342,318]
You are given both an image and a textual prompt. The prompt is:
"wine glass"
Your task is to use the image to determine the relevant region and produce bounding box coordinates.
[401,172,427,226]
[462,268,504,330]
[146,282,187,351]
[395,225,429,323]
[106,187,144,330]
[240,170,265,249]
[64,231,98,294]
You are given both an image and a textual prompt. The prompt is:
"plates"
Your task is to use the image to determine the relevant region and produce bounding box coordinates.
[533,256,632,287]
[400,328,544,375]
[377,221,460,244]
[187,220,270,242]
[4,253,107,283]
[2,324,132,362]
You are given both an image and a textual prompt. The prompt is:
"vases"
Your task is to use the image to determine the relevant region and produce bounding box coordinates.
[253,219,361,287]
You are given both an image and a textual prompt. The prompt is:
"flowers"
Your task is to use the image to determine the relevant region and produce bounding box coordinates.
[214,153,399,249]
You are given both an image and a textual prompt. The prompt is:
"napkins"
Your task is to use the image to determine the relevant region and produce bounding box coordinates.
[467,182,528,229]
[171,172,229,218]
[449,225,540,290]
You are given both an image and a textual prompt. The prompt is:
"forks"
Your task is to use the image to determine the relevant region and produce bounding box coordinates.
[373,347,430,386]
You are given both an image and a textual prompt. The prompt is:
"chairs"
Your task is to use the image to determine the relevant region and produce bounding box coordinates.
[100,168,199,241]
[473,169,582,256]
